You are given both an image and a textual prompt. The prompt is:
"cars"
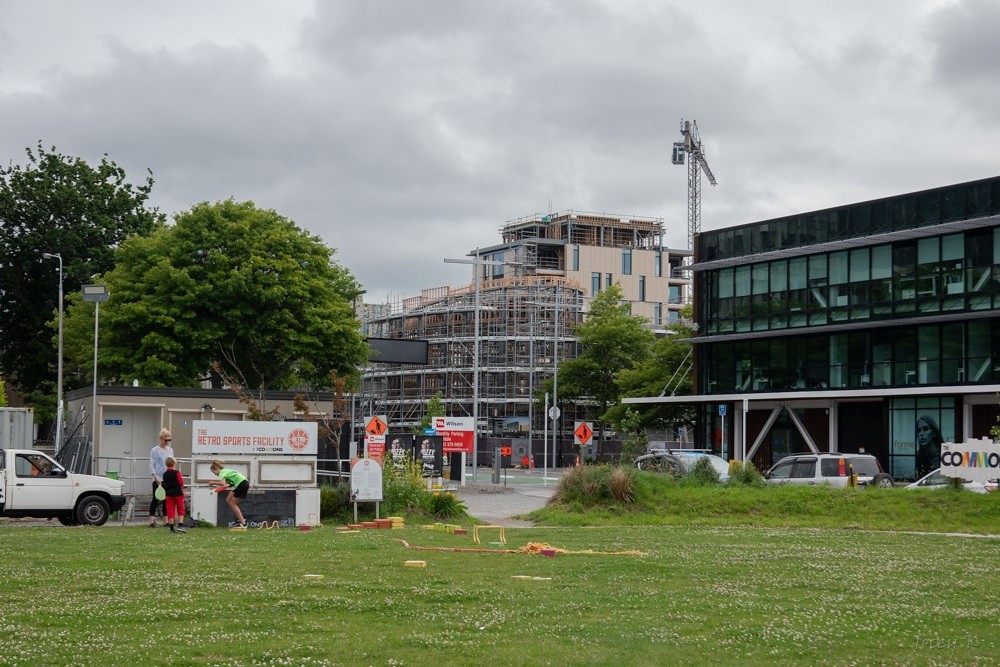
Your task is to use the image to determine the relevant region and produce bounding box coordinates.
[629,449,736,485]
[900,466,1000,496]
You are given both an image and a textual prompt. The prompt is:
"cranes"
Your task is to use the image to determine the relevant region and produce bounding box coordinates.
[669,118,718,304]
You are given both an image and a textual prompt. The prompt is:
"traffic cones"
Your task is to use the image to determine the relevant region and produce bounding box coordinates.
[531,455,535,469]
[575,456,581,468]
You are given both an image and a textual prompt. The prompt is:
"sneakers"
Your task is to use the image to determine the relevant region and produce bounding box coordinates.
[176,526,188,532]
[170,529,177,533]
[233,518,247,529]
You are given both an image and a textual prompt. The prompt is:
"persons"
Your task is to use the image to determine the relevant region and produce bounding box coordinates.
[391,438,404,458]
[915,415,943,479]
[209,460,249,529]
[163,457,188,533]
[148,428,174,527]
[586,449,595,463]
[421,438,437,470]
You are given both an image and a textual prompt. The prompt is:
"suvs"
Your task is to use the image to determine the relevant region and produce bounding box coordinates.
[760,451,895,493]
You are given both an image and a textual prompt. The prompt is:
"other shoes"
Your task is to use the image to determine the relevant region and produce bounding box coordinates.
[149,523,156,528]
[164,523,170,527]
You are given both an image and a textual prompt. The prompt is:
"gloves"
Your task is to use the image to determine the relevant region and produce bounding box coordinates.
[215,486,226,492]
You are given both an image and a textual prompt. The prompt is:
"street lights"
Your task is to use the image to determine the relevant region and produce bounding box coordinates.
[472,246,523,482]
[43,253,63,459]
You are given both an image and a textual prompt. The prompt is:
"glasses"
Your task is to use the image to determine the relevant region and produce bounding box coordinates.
[163,438,171,442]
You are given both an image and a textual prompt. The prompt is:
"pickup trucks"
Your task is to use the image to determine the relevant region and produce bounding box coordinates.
[0,448,128,527]
